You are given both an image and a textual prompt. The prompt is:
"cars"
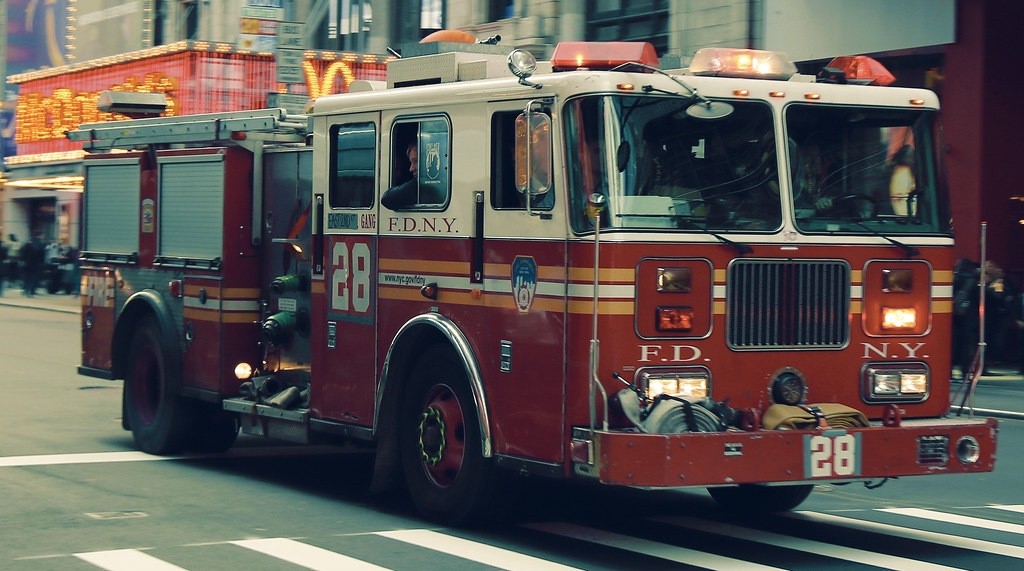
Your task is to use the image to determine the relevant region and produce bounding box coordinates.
[1,234,80,295]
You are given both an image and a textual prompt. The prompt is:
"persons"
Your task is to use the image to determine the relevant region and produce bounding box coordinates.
[0,229,46,295]
[887,144,918,219]
[538,134,608,234]
[380,142,418,212]
[772,143,844,217]
[952,258,1006,380]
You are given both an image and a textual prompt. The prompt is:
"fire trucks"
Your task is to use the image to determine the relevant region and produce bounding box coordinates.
[62,28,999,530]
